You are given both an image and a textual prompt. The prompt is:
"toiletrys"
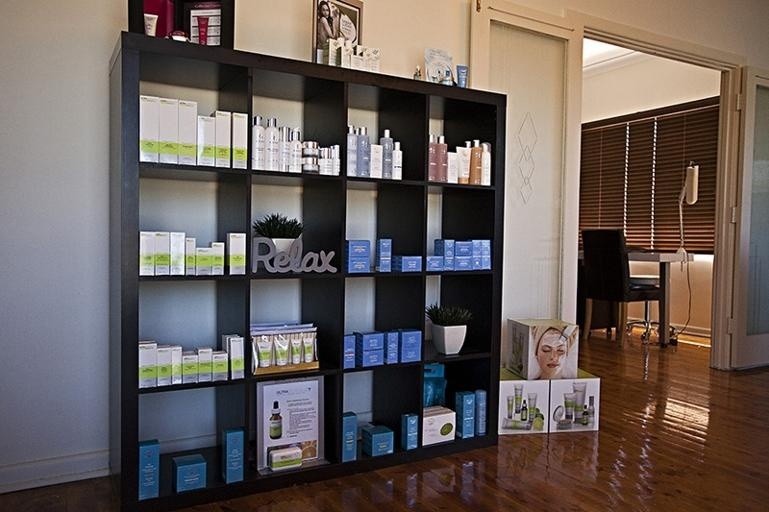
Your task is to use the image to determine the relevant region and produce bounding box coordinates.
[144,14,158,37]
[503,384,537,430]
[197,16,209,45]
[257,338,314,367]
[563,382,587,420]
[457,65,468,88]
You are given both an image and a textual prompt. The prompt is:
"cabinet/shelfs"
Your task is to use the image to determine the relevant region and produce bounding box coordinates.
[107,30,506,507]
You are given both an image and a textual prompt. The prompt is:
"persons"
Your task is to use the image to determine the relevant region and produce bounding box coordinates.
[530,324,577,380]
[317,1,342,48]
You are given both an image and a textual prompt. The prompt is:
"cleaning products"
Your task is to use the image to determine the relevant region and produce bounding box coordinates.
[347,124,402,180]
[252,115,340,177]
[428,133,491,186]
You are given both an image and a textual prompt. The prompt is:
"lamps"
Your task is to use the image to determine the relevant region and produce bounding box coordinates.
[676,160,701,253]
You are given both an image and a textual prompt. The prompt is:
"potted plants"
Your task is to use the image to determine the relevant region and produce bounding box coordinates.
[424,302,474,357]
[249,209,305,265]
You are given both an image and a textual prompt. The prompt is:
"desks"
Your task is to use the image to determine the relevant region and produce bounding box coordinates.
[575,247,687,347]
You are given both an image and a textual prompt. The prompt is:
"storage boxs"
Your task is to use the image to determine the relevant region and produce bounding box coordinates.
[507,317,580,380]
[498,366,603,436]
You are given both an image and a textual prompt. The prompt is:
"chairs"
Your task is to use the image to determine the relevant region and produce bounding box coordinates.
[585,226,663,347]
